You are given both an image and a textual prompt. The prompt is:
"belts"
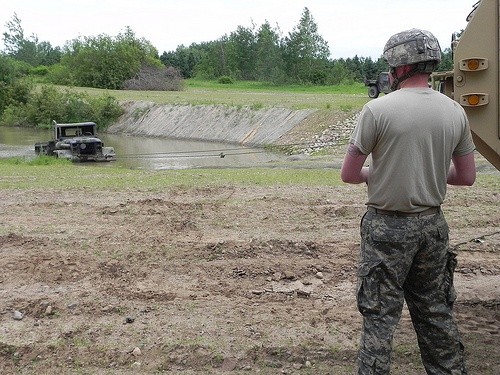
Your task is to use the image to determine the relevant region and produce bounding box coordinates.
[367,206,439,216]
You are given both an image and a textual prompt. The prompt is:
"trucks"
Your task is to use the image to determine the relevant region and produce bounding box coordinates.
[35,120,116,163]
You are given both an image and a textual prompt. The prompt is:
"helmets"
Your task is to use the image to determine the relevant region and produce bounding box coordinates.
[383,28,441,68]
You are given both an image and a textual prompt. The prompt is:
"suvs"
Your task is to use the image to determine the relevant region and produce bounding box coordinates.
[365,72,394,98]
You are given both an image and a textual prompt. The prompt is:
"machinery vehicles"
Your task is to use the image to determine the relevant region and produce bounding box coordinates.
[428,0,500,171]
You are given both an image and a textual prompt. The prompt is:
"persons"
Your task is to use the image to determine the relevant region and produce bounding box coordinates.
[340,27,477,375]
[75,128,91,137]
